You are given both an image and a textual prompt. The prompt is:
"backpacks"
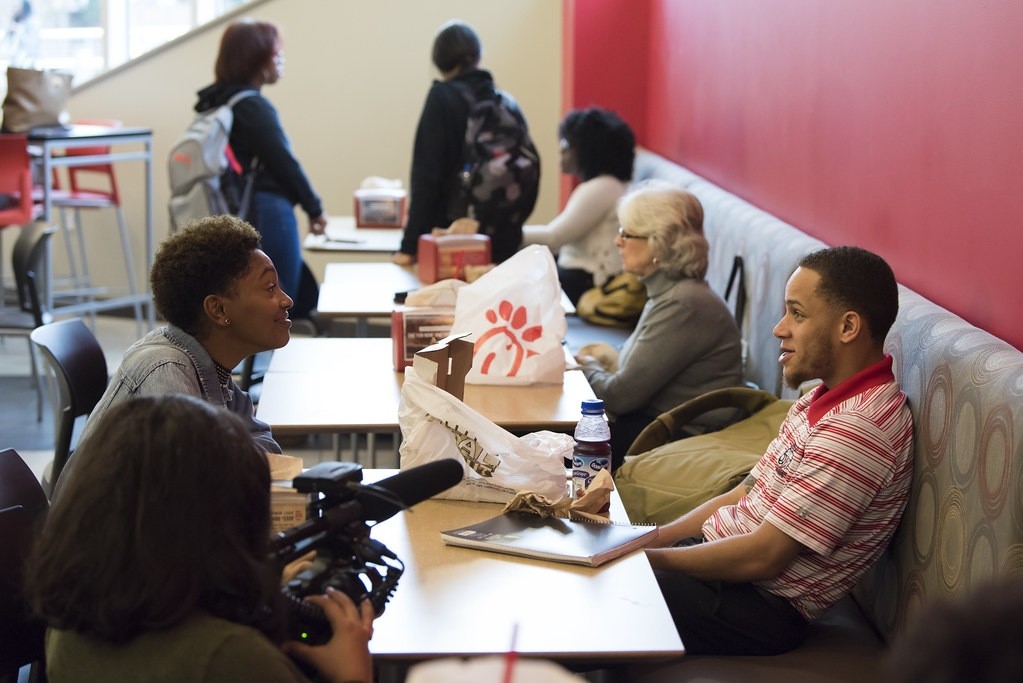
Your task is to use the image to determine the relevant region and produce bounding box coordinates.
[168,89,259,228]
[453,80,541,233]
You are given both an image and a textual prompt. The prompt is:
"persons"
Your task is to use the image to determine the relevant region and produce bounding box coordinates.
[541,246,915,673]
[193,20,328,318]
[522,107,635,305]
[76,216,294,454]
[392,21,527,267]
[18,402,374,683]
[573,181,744,472]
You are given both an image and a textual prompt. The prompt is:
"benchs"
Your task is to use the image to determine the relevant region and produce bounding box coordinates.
[565,146,1023,683]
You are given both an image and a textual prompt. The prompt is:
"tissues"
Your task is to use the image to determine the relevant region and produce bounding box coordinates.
[353,176,407,229]
[391,278,470,372]
[417,218,492,284]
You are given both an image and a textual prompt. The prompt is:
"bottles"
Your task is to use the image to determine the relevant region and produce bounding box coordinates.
[571,399,611,512]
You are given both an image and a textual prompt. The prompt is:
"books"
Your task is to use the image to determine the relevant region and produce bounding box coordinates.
[439,510,660,568]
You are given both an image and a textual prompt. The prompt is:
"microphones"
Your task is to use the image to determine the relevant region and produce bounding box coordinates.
[323,459,463,528]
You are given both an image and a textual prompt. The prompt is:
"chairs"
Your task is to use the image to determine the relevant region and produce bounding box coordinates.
[0,448,53,683]
[1,119,145,422]
[31,318,109,497]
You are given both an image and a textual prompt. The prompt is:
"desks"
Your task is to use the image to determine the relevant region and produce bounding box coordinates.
[256,337,610,469]
[319,260,577,336]
[318,467,687,683]
[304,216,404,253]
[25,123,153,329]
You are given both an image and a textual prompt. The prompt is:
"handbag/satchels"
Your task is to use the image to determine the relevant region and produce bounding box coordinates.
[577,271,647,328]
[397,365,577,504]
[3,68,73,133]
[453,244,576,386]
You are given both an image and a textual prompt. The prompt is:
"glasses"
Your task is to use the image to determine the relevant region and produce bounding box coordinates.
[619,227,649,240]
[558,138,571,153]
